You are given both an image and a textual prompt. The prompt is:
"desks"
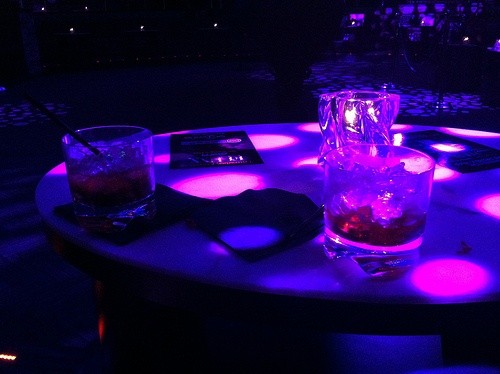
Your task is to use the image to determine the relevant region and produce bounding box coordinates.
[35,110,500,339]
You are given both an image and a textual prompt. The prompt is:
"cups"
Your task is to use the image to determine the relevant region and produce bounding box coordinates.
[321,142,436,272]
[315,88,401,171]
[62,125,158,225]
